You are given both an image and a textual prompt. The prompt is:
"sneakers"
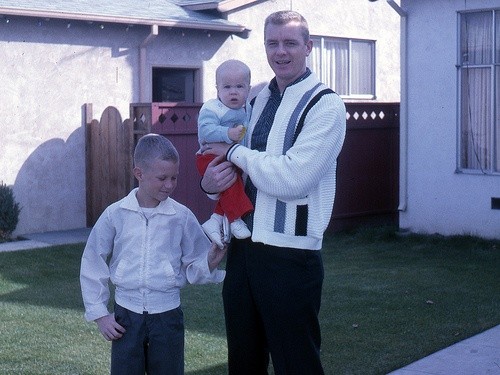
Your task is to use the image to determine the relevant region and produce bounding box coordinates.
[228,220,251,240]
[201,217,223,252]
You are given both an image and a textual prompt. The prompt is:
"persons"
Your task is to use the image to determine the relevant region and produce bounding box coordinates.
[201,10,347,375]
[79,133,227,375]
[195,59,252,250]
